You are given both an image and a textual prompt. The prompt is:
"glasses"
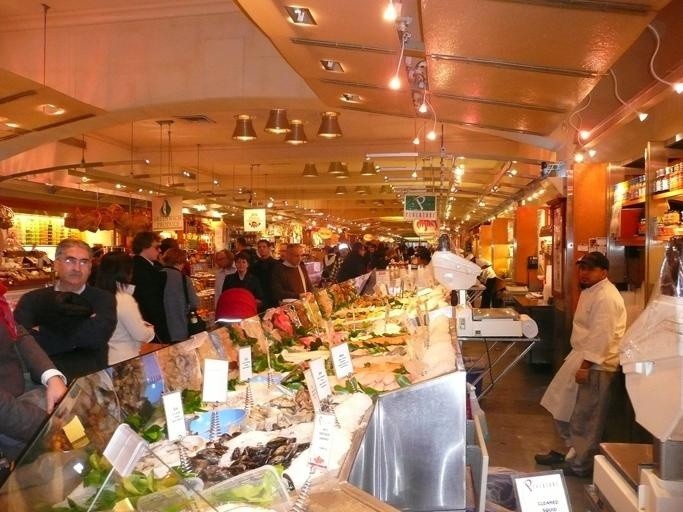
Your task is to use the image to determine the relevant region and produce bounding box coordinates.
[57,257,93,268]
[258,245,269,248]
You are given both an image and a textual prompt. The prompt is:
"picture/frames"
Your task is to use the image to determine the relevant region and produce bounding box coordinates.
[548,202,565,302]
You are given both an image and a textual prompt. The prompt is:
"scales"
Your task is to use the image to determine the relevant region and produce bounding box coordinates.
[432,251,523,338]
[593,295,683,512]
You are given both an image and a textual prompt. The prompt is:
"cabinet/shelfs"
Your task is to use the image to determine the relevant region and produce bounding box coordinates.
[646,140,681,243]
[601,151,645,246]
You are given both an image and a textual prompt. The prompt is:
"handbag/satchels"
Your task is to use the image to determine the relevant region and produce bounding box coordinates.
[187,307,207,334]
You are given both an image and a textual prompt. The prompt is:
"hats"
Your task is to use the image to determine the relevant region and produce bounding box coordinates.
[575,251,611,270]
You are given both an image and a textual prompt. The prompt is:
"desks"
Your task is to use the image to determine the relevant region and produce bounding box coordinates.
[512,294,554,369]
[455,335,539,400]
[453,286,485,303]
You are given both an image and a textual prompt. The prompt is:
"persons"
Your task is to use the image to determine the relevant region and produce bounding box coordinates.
[0,205,72,491]
[533,249,629,478]
[10,237,116,387]
[87,228,508,369]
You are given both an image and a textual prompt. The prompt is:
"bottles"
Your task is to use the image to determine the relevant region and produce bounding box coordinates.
[628,162,682,202]
[188,311,197,324]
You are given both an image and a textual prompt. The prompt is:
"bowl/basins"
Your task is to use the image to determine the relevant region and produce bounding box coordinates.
[189,409,247,439]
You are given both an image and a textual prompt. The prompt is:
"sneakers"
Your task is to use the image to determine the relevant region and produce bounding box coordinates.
[534,448,565,464]
[551,463,589,478]
